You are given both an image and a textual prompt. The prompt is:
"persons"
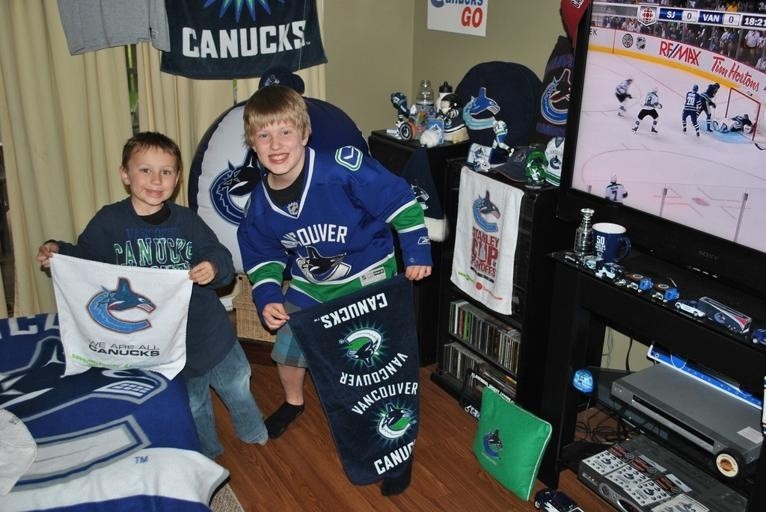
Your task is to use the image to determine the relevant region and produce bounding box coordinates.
[36,133,268,458]
[615,76,753,136]
[603,0,765,72]
[236,84,432,495]
[604,173,628,204]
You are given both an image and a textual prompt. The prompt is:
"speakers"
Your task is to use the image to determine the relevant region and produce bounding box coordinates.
[560,0,766,268]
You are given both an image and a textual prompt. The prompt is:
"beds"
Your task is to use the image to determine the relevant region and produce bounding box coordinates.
[0,312,231,512]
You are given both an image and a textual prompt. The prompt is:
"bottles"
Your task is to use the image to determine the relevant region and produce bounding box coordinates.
[416,79,453,114]
[574,207,596,254]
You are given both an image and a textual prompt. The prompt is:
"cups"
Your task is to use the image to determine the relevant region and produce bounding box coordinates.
[428,118,445,144]
[592,222,633,265]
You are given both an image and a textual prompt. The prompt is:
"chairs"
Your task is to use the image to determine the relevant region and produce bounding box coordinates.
[187,97,368,364]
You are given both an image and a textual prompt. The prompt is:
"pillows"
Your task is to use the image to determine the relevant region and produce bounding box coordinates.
[472,387,553,504]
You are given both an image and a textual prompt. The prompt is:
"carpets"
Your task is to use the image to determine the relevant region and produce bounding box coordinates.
[209,483,245,512]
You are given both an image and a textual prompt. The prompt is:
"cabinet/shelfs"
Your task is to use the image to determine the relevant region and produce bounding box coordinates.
[429,154,621,425]
[516,190,766,510]
[368,129,487,370]
